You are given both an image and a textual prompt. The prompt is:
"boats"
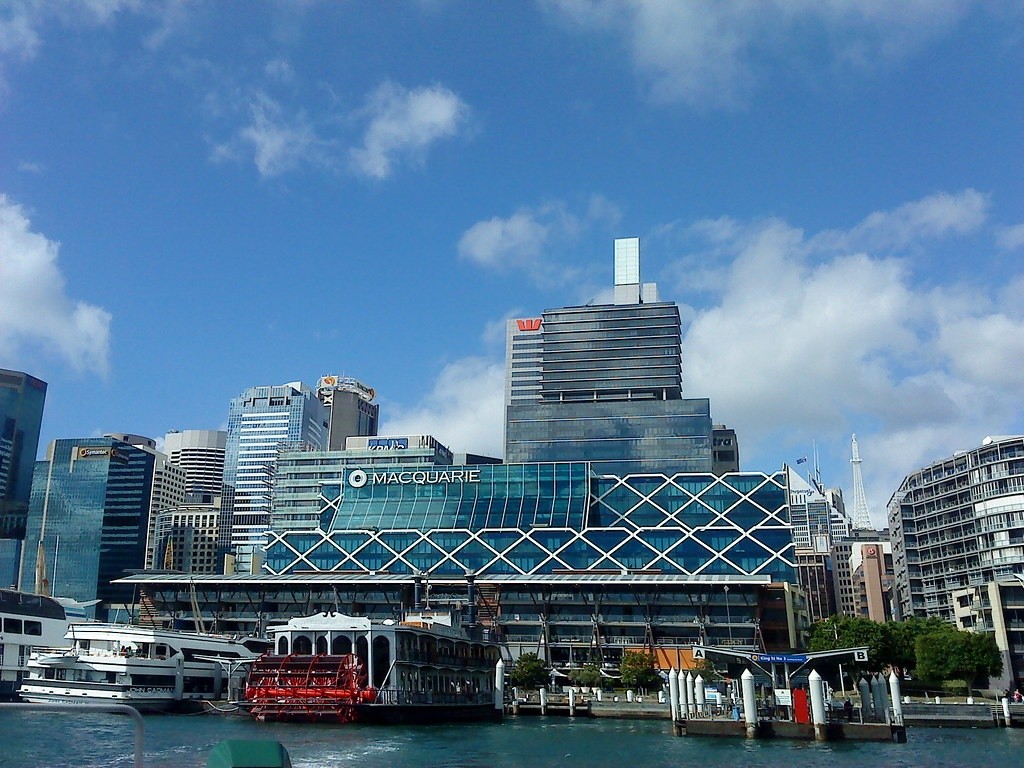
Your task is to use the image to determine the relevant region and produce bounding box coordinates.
[15,618,271,714]
[226,570,503,723]
[0,585,104,703]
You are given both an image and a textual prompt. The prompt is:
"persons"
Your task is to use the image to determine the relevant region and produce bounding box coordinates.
[1005,688,1024,704]
[136,645,142,657]
[843,698,854,722]
[121,646,132,655]
[790,683,808,724]
